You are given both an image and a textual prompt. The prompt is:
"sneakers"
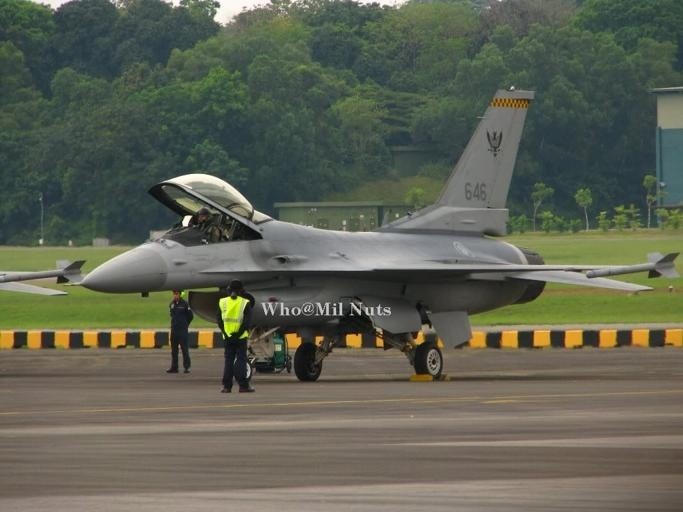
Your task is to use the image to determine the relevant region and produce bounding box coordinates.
[166,368,191,373]
[220,388,231,393]
[239,387,256,392]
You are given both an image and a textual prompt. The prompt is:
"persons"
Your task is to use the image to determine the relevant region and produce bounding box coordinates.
[164,290,193,374]
[170,207,210,245]
[213,278,256,393]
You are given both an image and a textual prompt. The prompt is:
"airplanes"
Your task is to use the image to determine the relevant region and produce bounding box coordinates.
[64,85,681,378]
[1,258,87,297]
